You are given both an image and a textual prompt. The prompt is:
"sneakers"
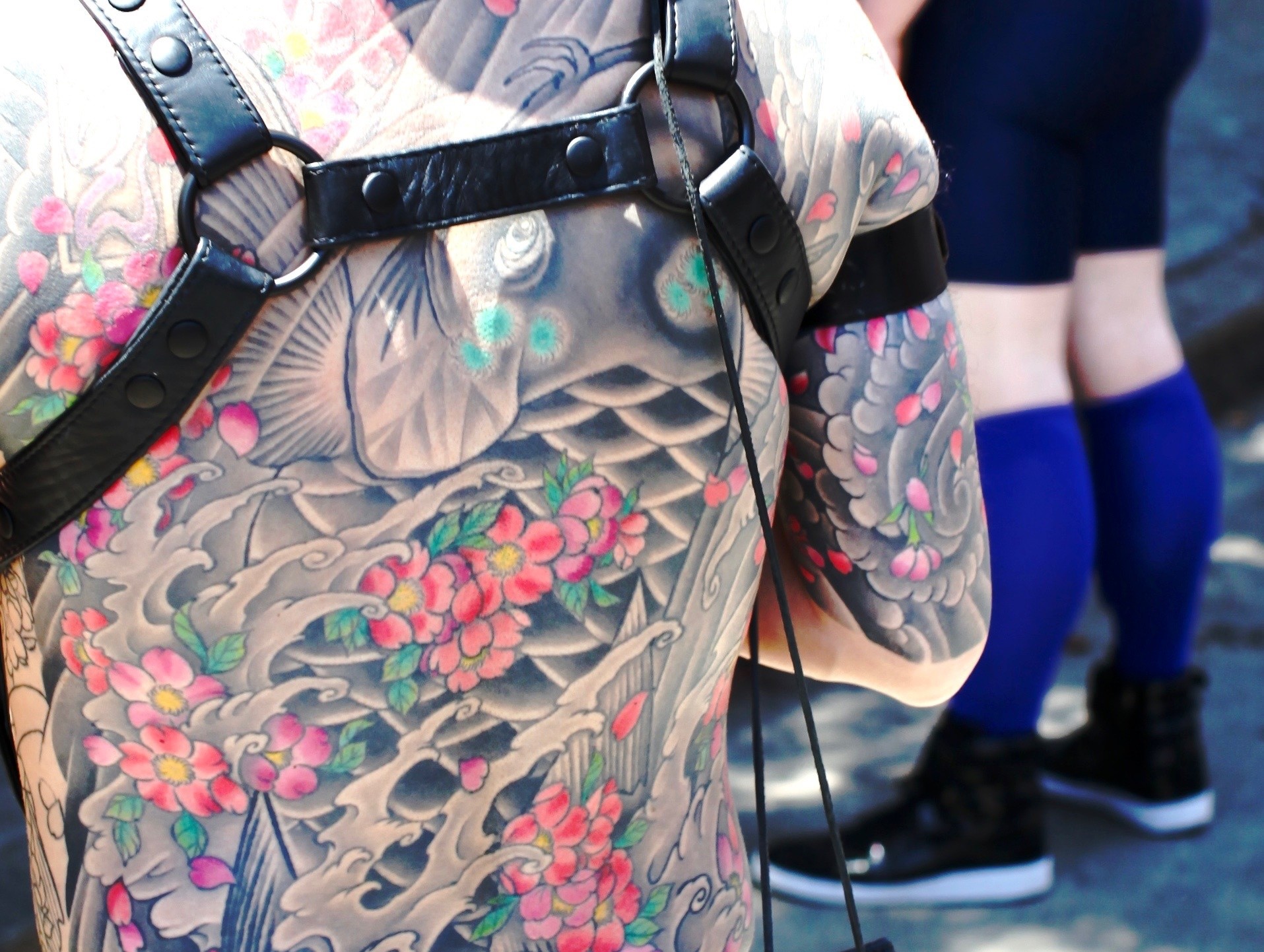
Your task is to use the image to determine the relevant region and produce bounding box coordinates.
[747,720,1056,911]
[1033,657,1216,838]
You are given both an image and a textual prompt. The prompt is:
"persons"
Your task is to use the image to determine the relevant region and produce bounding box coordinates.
[1,0,992,952]
[726,0,1229,910]
